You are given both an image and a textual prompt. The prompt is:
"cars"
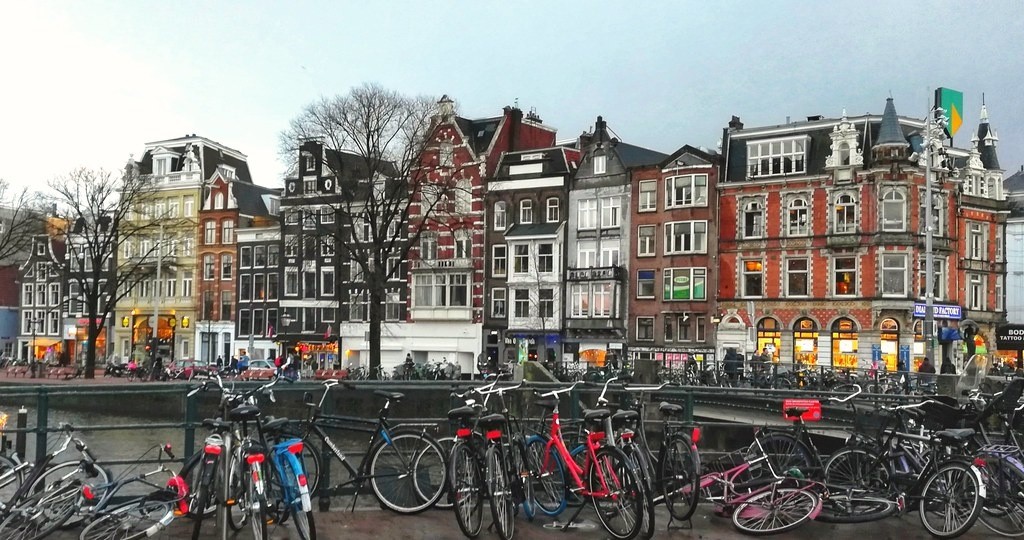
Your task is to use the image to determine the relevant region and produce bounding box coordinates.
[169,358,218,381]
[245,359,279,382]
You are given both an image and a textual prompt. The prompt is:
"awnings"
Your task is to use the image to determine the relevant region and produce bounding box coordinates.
[25,338,62,347]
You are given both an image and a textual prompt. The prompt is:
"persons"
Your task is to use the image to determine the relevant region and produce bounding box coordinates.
[218,350,250,382]
[154,353,162,380]
[272,349,300,385]
[723,346,738,386]
[406,352,414,361]
[919,357,956,384]
[751,348,771,374]
[58,350,66,367]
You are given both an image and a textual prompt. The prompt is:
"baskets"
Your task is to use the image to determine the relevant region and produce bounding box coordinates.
[853,413,890,439]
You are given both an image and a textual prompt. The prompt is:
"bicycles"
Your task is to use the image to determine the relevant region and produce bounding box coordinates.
[0,352,1024,539]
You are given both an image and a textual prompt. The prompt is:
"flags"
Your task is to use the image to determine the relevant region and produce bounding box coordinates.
[268,322,274,339]
[323,324,337,340]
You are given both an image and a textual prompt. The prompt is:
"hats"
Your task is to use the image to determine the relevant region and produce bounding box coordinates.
[924,357,929,361]
